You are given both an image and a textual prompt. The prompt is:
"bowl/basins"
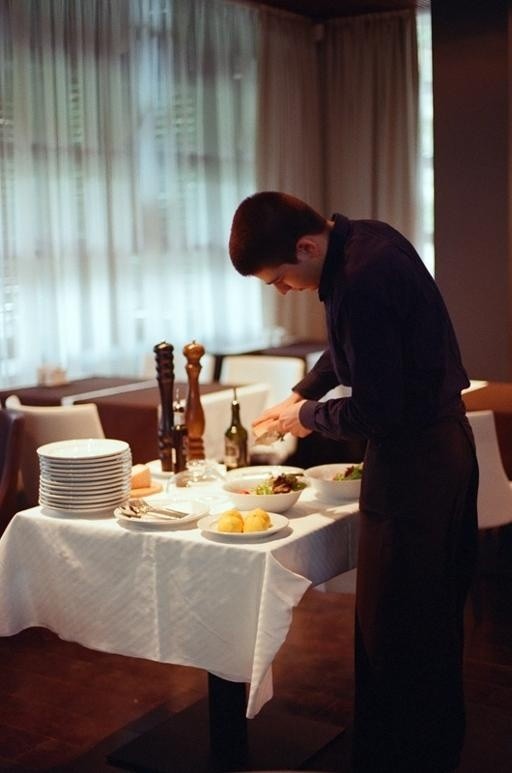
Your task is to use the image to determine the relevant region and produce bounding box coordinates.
[303,464,362,502]
[221,478,312,516]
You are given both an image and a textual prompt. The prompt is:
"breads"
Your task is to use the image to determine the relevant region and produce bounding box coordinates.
[131,463,151,489]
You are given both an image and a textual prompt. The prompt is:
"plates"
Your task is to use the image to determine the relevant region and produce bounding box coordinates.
[114,496,206,527]
[37,439,130,517]
[198,512,291,538]
[222,466,305,478]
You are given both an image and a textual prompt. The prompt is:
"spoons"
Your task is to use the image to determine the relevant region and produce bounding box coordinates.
[119,507,187,521]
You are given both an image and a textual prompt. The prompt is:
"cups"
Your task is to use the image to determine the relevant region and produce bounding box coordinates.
[185,459,207,482]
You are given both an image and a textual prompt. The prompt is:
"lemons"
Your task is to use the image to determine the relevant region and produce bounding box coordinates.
[217,508,270,533]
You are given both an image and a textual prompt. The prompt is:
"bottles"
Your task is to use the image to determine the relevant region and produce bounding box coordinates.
[170,406,189,473]
[223,401,249,469]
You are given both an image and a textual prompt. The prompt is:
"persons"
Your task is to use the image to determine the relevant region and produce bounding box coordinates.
[228,189,481,773]
